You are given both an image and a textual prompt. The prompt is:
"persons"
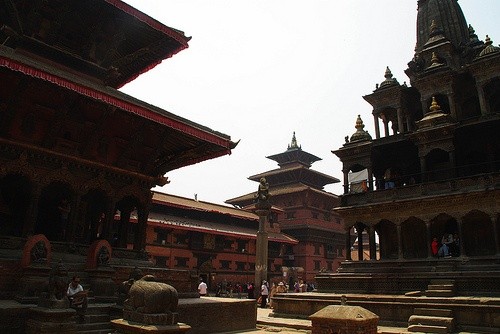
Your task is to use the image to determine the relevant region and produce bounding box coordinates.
[431,231,460,260]
[67,276,89,322]
[197,278,208,297]
[217,279,317,309]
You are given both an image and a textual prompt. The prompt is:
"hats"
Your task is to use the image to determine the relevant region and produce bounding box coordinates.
[262,280,267,284]
[278,282,284,285]
[73,276,80,281]
